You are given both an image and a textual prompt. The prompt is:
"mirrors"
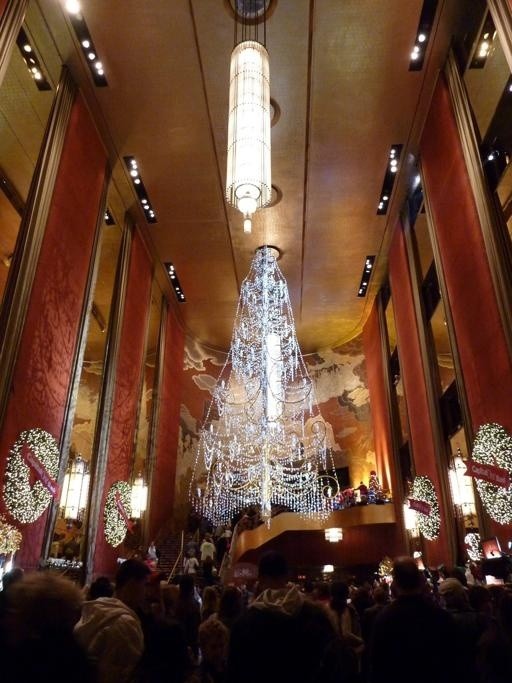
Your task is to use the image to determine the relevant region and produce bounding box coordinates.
[0,0,82,430]
[371,261,433,564]
[395,149,490,587]
[441,0,511,355]
[117,262,172,587]
[41,164,135,609]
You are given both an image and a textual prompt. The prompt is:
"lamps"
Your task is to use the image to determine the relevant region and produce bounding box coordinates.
[184,243,346,535]
[321,525,344,543]
[399,481,420,541]
[220,0,281,234]
[443,444,479,528]
[59,450,92,532]
[129,468,151,527]
